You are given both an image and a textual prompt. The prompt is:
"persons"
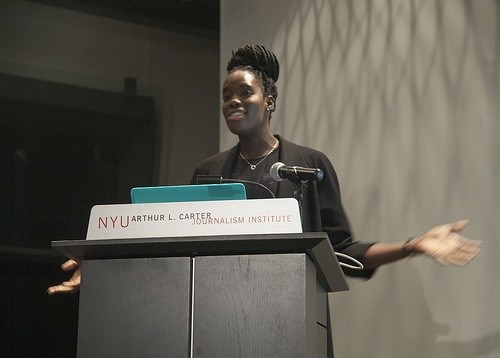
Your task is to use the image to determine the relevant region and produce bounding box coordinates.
[47,43,483,297]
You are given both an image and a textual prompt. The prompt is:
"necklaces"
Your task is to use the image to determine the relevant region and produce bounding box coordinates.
[238,137,280,171]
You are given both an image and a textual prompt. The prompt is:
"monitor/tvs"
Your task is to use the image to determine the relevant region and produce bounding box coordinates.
[129,182,248,204]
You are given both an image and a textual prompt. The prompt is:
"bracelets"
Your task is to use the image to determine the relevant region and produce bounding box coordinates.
[400,234,417,259]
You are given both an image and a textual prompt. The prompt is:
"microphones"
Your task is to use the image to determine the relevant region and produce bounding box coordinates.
[195,174,276,199]
[269,161,324,183]
